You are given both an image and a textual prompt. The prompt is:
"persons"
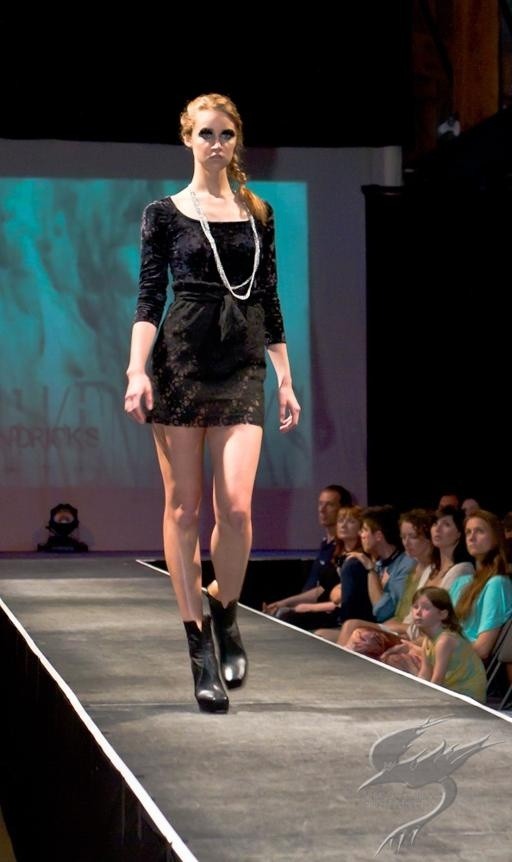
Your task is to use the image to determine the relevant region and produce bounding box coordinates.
[123,93,300,712]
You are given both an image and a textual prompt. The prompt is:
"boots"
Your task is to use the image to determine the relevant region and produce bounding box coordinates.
[182,588,247,715]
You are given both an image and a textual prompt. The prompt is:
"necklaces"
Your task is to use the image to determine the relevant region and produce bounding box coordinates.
[187,183,261,301]
